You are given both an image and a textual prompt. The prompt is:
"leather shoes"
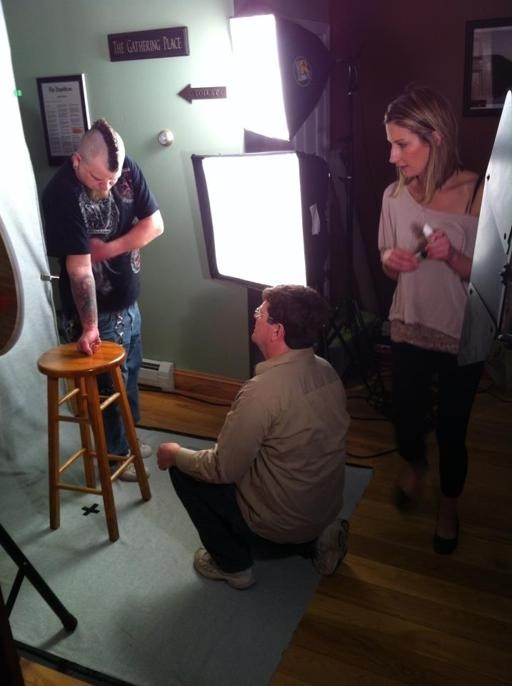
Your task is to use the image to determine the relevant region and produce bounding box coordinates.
[392,482,414,514]
[434,506,460,553]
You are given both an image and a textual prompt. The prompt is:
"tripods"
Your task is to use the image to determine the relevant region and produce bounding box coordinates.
[316,62,390,416]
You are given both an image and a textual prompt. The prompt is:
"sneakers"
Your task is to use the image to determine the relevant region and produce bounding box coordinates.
[111,459,150,481]
[130,441,151,457]
[193,545,253,589]
[313,518,349,575]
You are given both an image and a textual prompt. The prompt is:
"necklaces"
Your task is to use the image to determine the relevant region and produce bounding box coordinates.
[92,192,111,230]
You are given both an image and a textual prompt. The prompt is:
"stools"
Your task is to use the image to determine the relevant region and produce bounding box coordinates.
[35,341,152,544]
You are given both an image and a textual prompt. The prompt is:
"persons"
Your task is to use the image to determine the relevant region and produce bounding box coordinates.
[379,88,483,555]
[157,285,351,590]
[40,118,164,481]
